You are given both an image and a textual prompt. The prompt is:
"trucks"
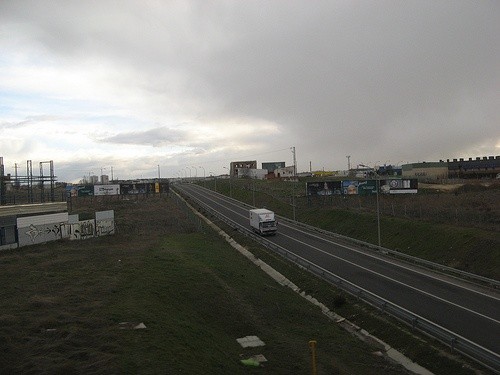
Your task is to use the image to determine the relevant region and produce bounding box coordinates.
[249,208,278,237]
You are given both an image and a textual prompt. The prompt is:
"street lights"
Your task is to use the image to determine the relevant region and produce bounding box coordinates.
[359,164,381,250]
[88,166,114,182]
[175,166,232,198]
